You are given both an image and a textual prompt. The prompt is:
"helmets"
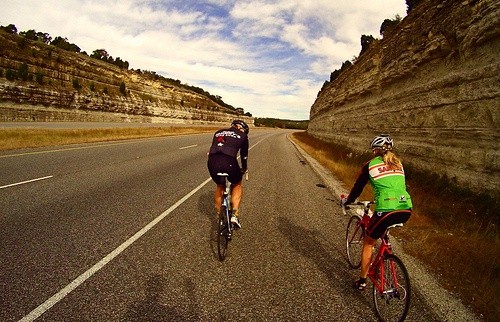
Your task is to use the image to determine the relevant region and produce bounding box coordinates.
[232,119,249,134]
[370,135,394,151]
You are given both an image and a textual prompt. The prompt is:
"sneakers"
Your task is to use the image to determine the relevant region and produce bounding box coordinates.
[231,217,241,229]
[354,280,366,292]
[384,239,392,246]
[217,219,223,226]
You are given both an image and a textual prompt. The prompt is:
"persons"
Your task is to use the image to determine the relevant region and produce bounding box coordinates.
[207,120,249,228]
[339,134,413,290]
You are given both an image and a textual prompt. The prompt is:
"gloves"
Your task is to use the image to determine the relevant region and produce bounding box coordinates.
[340,199,350,210]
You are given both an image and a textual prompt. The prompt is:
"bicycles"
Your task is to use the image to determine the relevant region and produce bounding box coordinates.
[213,167,250,261]
[340,193,411,322]
